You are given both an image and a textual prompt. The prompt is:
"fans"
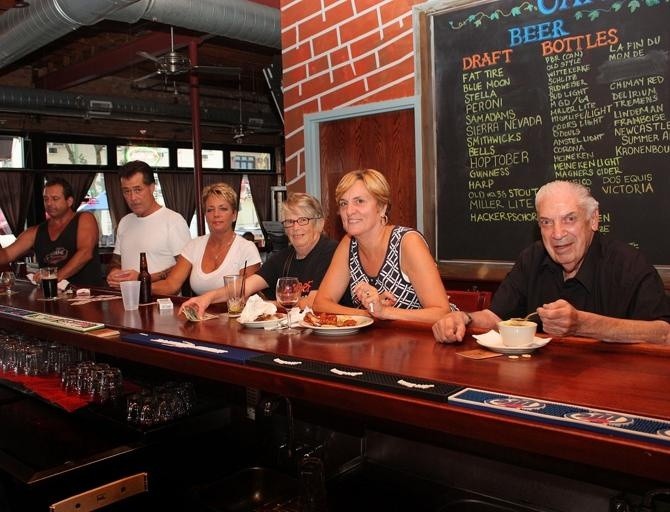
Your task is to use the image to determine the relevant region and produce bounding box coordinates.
[130,26,243,90]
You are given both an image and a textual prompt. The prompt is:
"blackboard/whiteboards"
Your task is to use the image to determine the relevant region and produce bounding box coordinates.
[419,0,670,289]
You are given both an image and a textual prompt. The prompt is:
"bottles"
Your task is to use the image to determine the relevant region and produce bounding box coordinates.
[136,253,154,304]
[139,306,155,332]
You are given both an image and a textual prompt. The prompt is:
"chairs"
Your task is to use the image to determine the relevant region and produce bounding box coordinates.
[446,288,493,313]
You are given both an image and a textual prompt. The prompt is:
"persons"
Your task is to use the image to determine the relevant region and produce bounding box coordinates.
[177,192,352,320]
[311,168,462,324]
[106,160,191,297]
[432,179,670,344]
[151,183,261,304]
[0,177,106,290]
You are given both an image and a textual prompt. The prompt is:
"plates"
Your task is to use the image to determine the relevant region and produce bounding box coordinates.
[236,312,286,329]
[298,314,374,337]
[474,334,554,355]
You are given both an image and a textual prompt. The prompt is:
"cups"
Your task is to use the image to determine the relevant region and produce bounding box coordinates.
[0,331,126,406]
[38,267,60,298]
[496,320,539,347]
[123,310,142,331]
[118,280,142,310]
[43,299,58,314]
[128,379,200,426]
[222,274,246,317]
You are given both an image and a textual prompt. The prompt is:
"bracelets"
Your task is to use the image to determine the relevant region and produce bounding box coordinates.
[465,312,472,328]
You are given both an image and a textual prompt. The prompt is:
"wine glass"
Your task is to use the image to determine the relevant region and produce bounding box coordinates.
[0,271,17,294]
[275,276,300,336]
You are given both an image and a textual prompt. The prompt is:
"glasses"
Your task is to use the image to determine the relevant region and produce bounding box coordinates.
[279,216,322,227]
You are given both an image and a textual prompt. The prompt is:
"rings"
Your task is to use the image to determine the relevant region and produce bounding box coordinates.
[366,292,369,296]
